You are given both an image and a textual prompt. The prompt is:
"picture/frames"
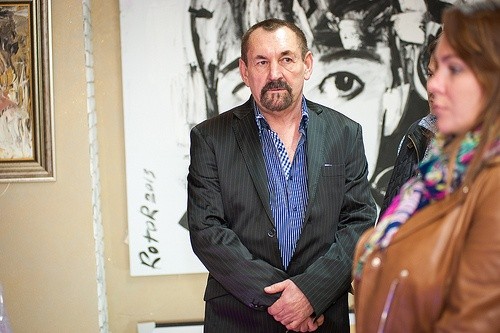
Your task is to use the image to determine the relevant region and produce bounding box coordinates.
[1,0,58,183]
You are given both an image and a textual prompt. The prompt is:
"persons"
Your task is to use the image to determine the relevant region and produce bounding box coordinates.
[184,18,376,333]
[377,38,469,228]
[355,0,499,333]
